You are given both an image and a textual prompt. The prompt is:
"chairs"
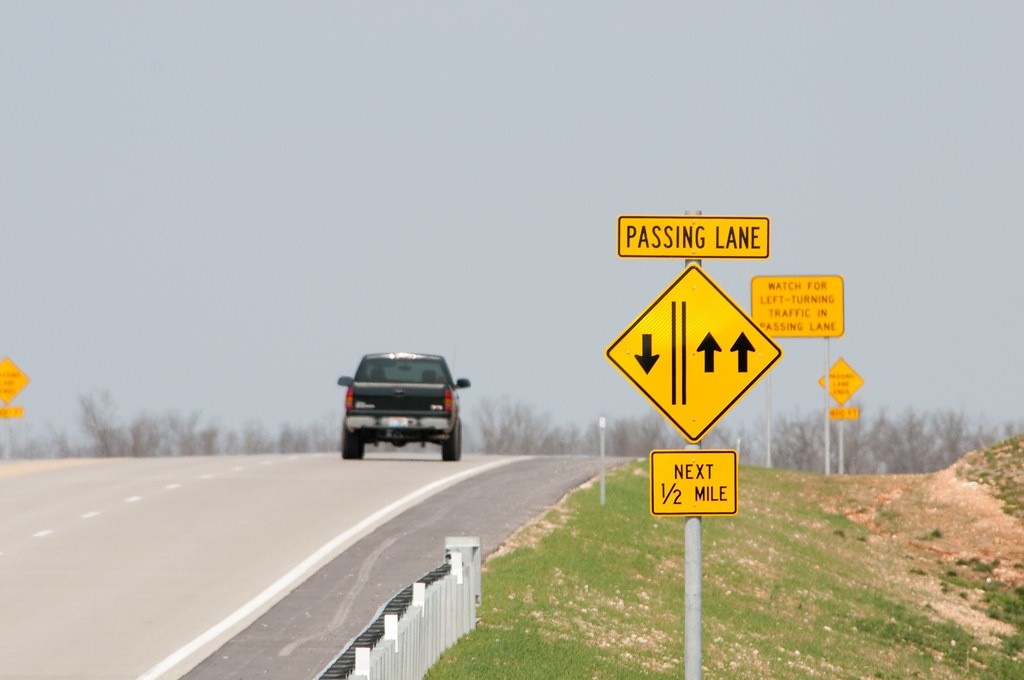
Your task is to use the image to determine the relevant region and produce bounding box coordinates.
[371,366,386,381]
[422,370,438,383]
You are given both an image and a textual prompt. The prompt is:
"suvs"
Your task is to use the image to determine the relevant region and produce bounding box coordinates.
[337,352,471,461]
